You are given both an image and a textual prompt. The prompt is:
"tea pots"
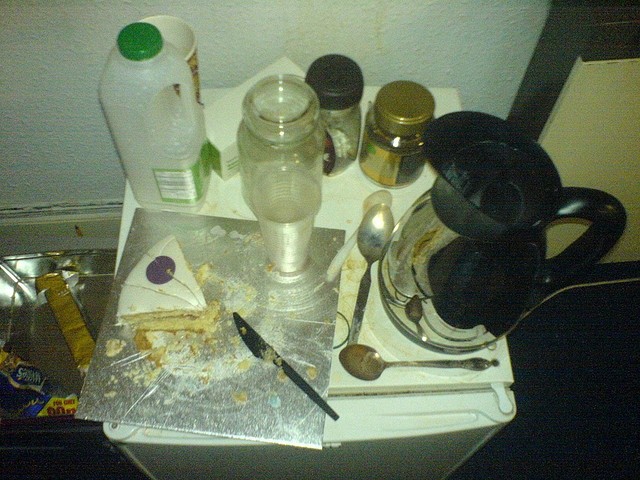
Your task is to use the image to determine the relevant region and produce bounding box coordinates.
[376,112,627,355]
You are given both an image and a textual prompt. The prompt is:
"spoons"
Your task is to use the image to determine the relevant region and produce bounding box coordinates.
[339,344,493,381]
[326,190,392,282]
[404,295,431,344]
[347,203,394,344]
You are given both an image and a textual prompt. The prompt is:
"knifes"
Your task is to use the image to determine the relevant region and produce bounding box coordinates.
[233,311,340,422]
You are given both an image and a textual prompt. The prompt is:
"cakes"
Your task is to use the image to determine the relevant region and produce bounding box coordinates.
[115,234,217,336]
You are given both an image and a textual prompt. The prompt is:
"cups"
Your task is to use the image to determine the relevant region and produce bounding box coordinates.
[136,16,199,106]
[253,165,322,273]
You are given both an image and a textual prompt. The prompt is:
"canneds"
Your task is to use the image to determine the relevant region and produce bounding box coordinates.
[358,80,434,188]
